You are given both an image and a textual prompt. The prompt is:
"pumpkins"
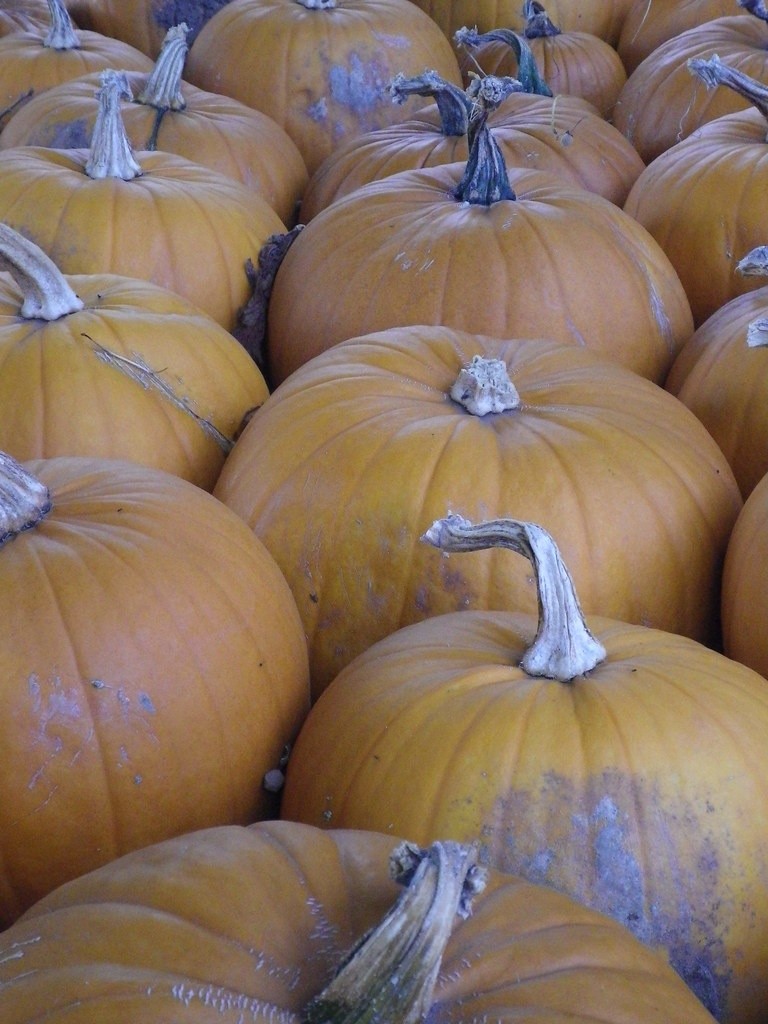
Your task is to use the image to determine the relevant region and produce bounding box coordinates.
[0,0,768,1024]
